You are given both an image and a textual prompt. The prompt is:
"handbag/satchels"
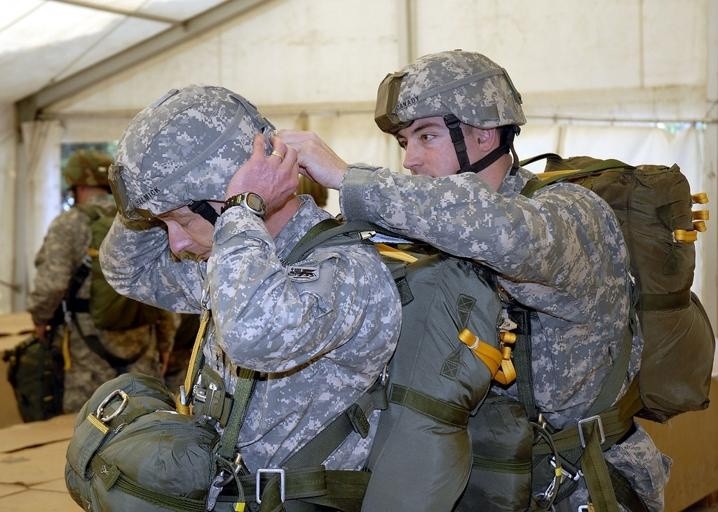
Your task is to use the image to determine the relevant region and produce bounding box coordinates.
[451,395,535,512]
[8,337,65,424]
[65,373,220,512]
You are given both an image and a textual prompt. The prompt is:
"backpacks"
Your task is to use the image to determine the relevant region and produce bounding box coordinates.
[72,203,160,332]
[281,218,502,512]
[484,153,716,454]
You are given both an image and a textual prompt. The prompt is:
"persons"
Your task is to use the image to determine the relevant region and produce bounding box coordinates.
[279,47,674,511]
[24,145,182,417]
[96,83,407,511]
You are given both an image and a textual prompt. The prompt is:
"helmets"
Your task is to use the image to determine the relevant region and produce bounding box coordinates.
[375,50,526,134]
[64,149,114,187]
[109,86,276,230]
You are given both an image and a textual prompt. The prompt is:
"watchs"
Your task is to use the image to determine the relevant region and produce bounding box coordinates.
[221,192,267,218]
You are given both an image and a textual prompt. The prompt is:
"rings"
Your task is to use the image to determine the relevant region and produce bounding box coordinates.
[271,150,285,161]
